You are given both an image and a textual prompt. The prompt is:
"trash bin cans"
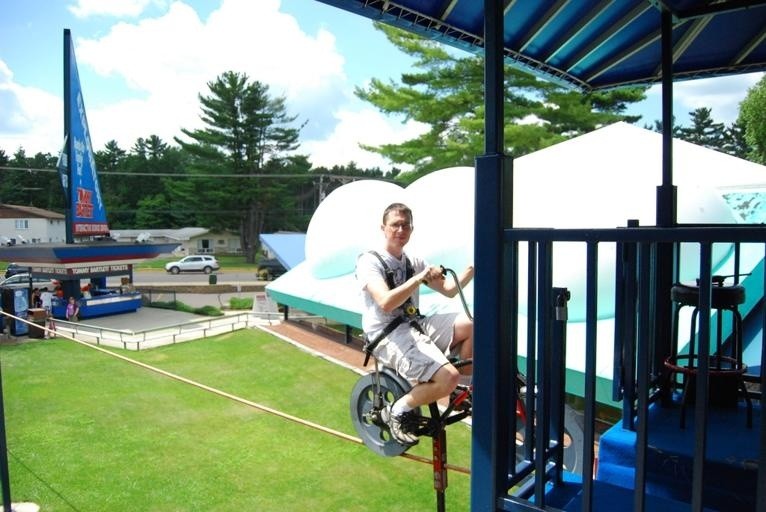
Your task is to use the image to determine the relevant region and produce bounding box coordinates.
[27,308,46,338]
[210,274,217,283]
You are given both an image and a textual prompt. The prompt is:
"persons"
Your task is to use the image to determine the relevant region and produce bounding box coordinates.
[120,278,135,295]
[65,296,80,335]
[353,202,474,448]
[79,278,99,298]
[30,280,64,331]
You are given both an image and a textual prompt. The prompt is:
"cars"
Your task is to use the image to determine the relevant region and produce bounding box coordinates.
[165,254,220,274]
[255,258,287,281]
[4,263,31,279]
[0,273,58,292]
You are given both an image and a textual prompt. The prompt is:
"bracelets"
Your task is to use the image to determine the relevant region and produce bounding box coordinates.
[466,266,473,271]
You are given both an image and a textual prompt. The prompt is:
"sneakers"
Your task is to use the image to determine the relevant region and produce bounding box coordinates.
[380,405,419,447]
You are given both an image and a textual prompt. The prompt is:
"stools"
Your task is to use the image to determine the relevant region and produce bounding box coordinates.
[663,280,755,433]
